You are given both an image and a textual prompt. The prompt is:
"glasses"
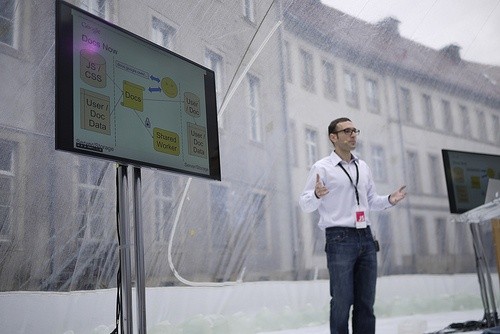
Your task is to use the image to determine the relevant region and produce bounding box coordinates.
[333,127,361,136]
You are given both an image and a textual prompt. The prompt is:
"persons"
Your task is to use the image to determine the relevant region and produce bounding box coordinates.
[298,117,408,334]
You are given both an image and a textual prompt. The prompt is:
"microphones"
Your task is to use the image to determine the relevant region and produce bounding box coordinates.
[344,141,347,144]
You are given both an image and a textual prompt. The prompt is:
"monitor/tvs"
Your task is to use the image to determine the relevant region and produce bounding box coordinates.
[55,0,221,181]
[442,149,500,214]
